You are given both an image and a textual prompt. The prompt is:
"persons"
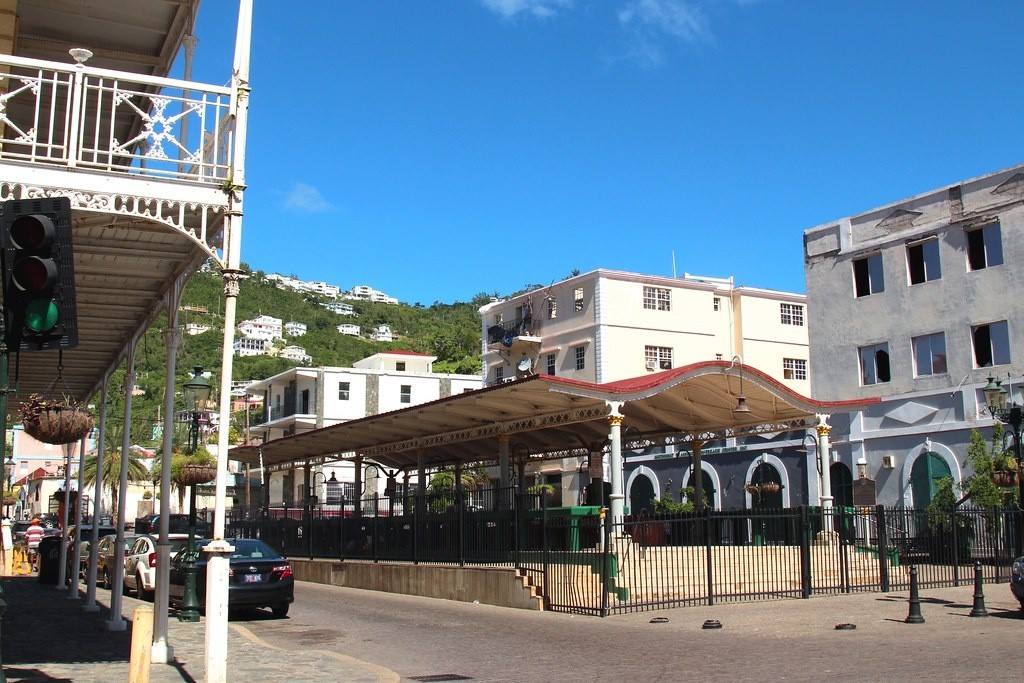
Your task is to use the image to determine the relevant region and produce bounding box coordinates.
[25,518,44,572]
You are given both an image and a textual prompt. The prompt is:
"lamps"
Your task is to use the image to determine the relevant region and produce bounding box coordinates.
[361,465,381,494]
[578,460,589,474]
[672,450,695,480]
[426,455,447,490]
[329,454,338,481]
[315,472,328,485]
[510,442,532,480]
[725,355,751,412]
[622,426,643,464]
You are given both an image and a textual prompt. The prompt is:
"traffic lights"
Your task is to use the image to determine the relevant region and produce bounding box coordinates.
[4,197,79,352]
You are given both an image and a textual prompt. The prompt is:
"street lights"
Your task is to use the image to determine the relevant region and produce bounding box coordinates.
[3,456,17,519]
[178,366,213,622]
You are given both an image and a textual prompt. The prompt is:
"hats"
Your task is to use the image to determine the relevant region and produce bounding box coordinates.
[33,518,40,523]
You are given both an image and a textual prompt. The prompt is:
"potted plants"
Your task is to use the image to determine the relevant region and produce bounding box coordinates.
[762,481,779,494]
[746,484,761,494]
[528,486,539,495]
[540,484,555,496]
[171,445,218,487]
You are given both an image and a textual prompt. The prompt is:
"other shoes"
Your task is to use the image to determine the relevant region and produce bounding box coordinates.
[34,565,38,572]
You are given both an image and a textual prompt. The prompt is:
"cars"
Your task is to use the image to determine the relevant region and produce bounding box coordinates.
[82,534,149,588]
[123,534,201,597]
[1010,551,1024,609]
[144,514,211,536]
[169,538,294,616]
[33,513,59,528]
[28,528,60,562]
[69,525,117,579]
[11,520,47,544]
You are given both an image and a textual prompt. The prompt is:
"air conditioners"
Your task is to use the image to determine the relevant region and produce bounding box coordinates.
[647,361,656,369]
